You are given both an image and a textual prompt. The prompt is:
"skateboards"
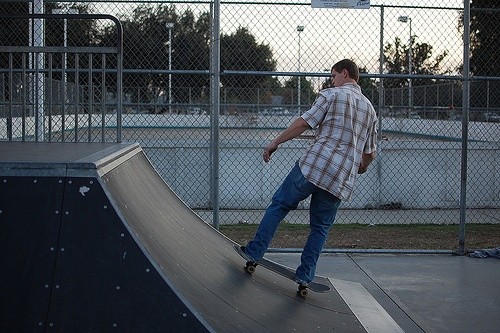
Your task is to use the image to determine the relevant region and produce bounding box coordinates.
[232,244,332,298]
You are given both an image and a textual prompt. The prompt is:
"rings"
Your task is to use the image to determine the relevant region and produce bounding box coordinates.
[263,152,266,157]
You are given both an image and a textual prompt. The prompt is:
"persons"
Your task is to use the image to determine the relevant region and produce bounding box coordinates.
[238,59,378,288]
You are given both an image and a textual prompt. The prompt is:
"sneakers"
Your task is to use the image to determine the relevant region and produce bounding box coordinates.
[294,276,309,288]
[233,244,260,265]
